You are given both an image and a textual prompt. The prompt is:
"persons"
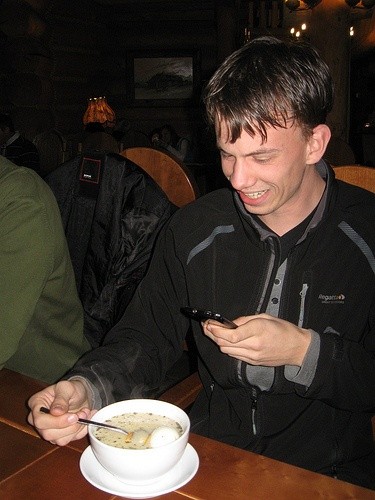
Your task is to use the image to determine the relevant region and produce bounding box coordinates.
[27,37,375,488]
[158,124,192,161]
[0,113,40,173]
[0,155,92,383]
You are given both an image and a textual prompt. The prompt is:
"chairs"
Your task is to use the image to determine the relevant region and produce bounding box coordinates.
[33,129,197,208]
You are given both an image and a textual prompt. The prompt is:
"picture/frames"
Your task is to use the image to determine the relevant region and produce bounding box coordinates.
[126,45,202,107]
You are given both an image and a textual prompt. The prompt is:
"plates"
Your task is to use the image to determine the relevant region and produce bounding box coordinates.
[79,442,199,499]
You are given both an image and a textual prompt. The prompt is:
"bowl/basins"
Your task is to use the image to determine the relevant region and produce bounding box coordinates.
[87,399,190,486]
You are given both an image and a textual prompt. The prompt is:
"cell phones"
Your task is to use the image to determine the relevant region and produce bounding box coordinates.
[180,307,239,329]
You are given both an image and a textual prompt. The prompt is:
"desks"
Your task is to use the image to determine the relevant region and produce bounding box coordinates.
[0,364,375,500]
[184,161,210,195]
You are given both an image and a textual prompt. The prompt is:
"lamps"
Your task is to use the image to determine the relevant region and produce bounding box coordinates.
[345,0,375,10]
[285,0,323,11]
[83,97,116,131]
